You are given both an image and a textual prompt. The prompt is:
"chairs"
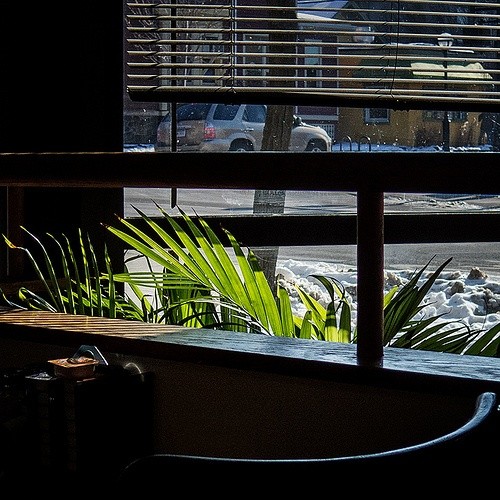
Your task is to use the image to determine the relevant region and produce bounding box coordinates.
[119,391,500,500]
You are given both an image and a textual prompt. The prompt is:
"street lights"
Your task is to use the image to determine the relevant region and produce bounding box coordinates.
[437,33,454,153]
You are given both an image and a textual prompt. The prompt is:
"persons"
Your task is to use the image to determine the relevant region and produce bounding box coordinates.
[478,112,493,145]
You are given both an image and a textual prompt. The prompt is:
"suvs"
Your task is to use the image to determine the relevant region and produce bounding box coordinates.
[156,99,333,152]
